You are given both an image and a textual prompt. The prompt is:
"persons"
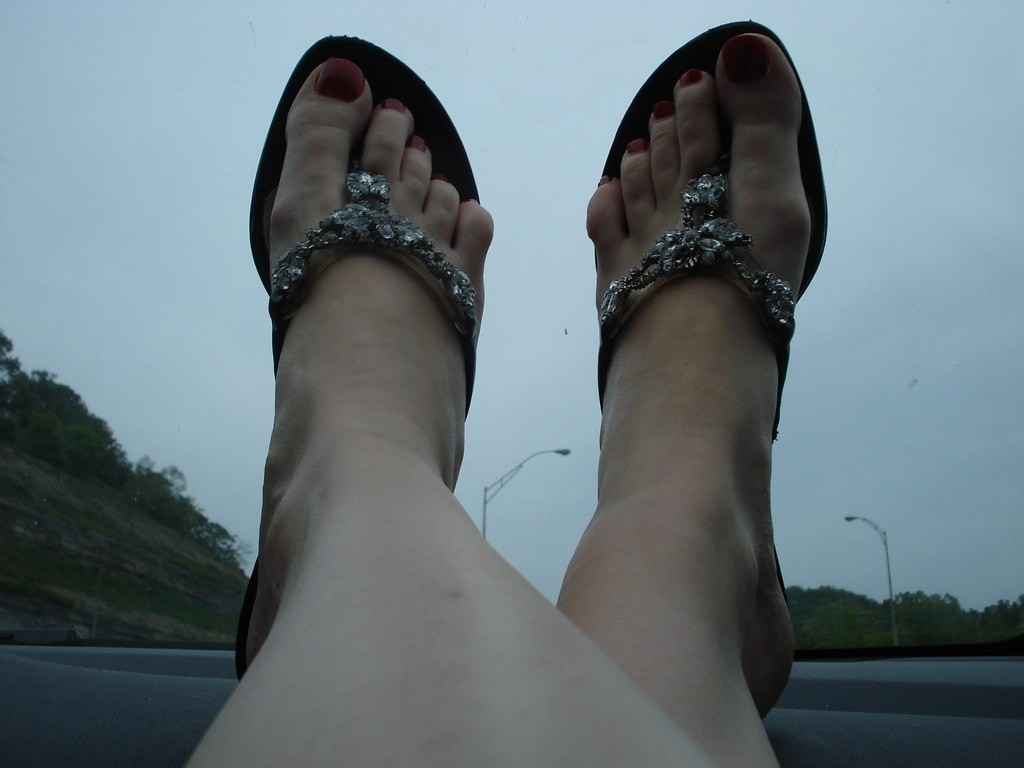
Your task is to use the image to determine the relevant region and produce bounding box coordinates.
[184,21,829,768]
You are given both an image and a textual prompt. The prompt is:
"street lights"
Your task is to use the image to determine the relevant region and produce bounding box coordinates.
[844,515,899,646]
[480,448,573,541]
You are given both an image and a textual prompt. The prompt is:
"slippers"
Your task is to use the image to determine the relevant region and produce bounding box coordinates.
[231,33,480,681]
[588,19,793,599]
